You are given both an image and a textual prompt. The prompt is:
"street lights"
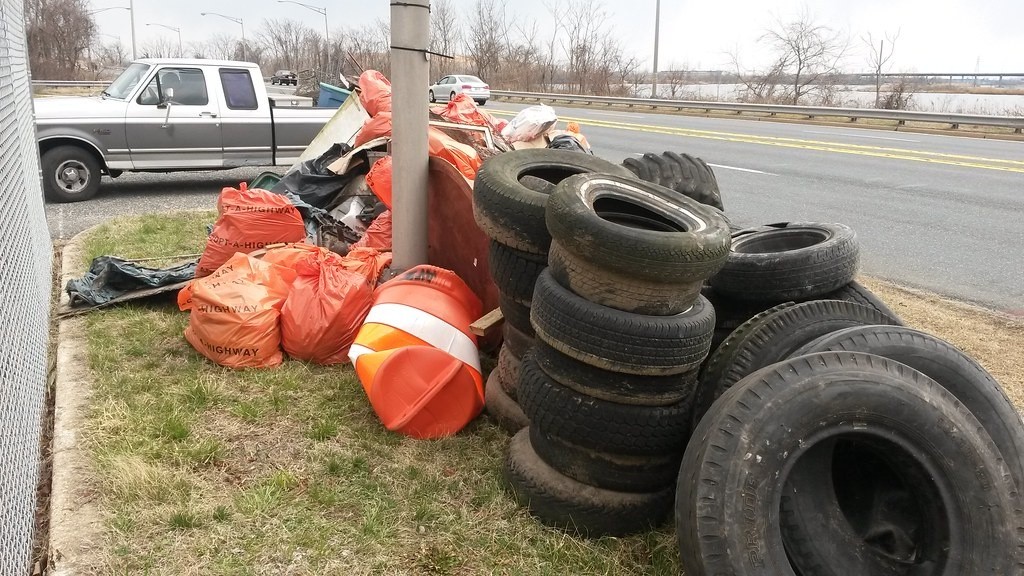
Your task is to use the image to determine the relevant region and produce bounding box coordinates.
[146,23,183,57]
[200,12,247,62]
[277,1,333,84]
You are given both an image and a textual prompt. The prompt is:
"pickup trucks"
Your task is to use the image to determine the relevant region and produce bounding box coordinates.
[33,58,340,203]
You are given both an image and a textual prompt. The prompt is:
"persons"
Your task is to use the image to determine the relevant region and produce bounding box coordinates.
[161,72,181,98]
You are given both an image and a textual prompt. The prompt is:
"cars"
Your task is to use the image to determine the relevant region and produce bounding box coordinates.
[429,75,490,105]
[269,70,297,86]
